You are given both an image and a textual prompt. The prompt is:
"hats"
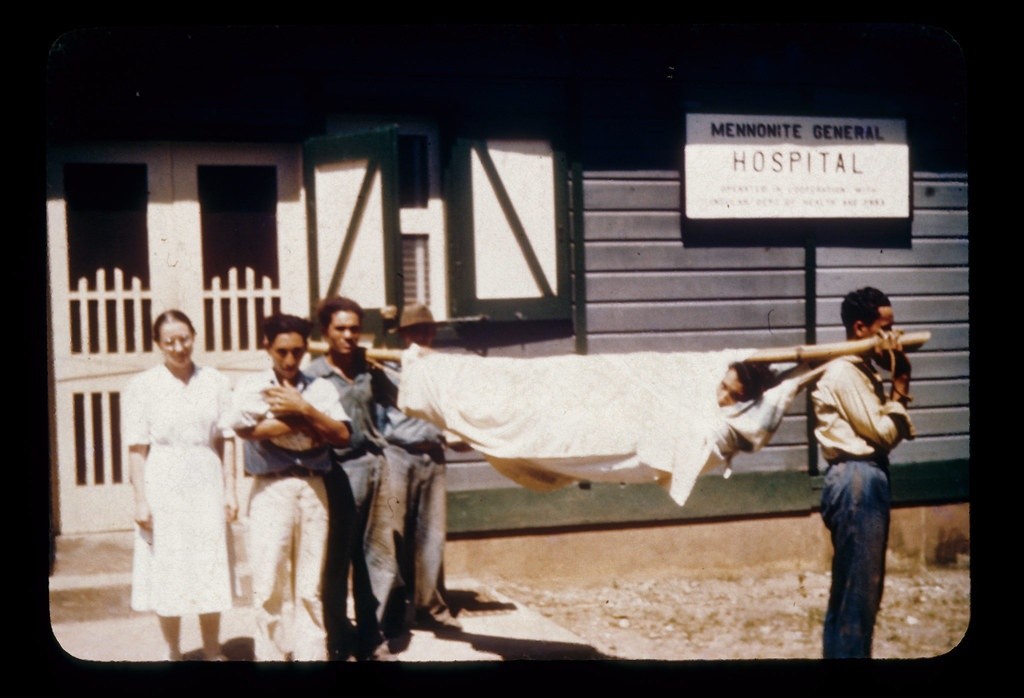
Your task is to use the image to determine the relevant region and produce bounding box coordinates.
[262,315,313,335]
[387,303,449,335]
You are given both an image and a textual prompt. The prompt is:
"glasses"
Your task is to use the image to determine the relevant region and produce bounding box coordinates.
[161,337,194,346]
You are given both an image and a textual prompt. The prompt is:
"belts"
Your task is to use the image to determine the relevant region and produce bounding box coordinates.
[264,467,321,477]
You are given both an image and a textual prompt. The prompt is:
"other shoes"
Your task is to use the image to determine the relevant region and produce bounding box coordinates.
[353,628,382,646]
[204,652,229,661]
[415,608,462,632]
[382,623,403,638]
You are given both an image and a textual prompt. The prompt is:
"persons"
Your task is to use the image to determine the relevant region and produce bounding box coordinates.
[121,297,473,663]
[716,361,776,407]
[812,287,917,659]
